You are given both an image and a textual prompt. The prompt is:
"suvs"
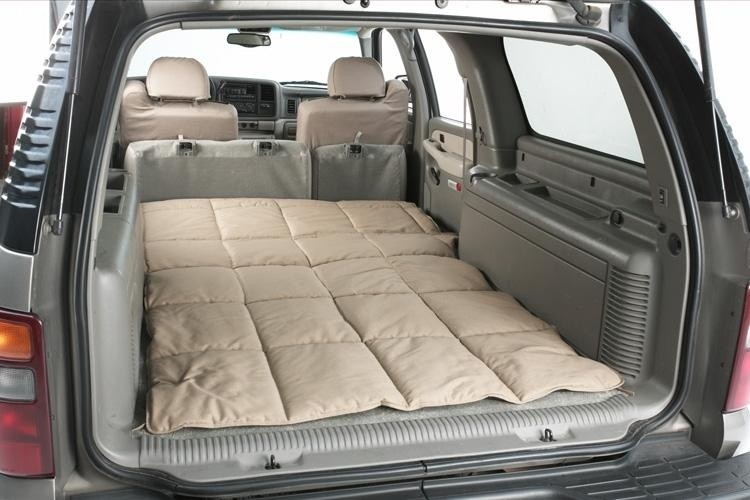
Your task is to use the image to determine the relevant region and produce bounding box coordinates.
[0,0,747,498]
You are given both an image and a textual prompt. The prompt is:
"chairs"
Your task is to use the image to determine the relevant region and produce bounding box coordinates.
[116,57,237,166]
[295,56,409,150]
